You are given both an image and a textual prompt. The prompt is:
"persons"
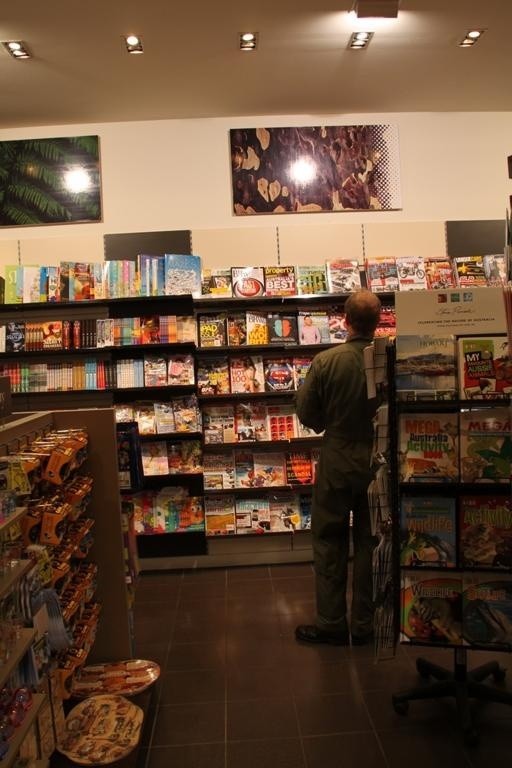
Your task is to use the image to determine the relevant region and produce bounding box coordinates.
[291,287,387,647]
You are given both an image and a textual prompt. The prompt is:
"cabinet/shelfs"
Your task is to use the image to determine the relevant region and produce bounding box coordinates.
[191,291,395,548]
[0,503,49,768]
[0,293,206,559]
[359,333,512,747]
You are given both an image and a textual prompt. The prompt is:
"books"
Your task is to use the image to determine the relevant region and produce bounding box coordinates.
[114,439,321,491]
[461,410,512,484]
[1,252,512,303]
[370,403,388,466]
[398,492,457,572]
[459,490,512,571]
[396,407,461,483]
[394,330,457,404]
[364,469,389,537]
[131,491,325,539]
[460,574,512,651]
[460,333,512,403]
[361,338,390,399]
[368,540,394,645]
[397,572,460,649]
[107,396,323,442]
[0,307,397,347]
[2,354,312,394]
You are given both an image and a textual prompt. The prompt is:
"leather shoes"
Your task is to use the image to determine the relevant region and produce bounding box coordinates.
[352,629,373,646]
[295,625,349,646]
